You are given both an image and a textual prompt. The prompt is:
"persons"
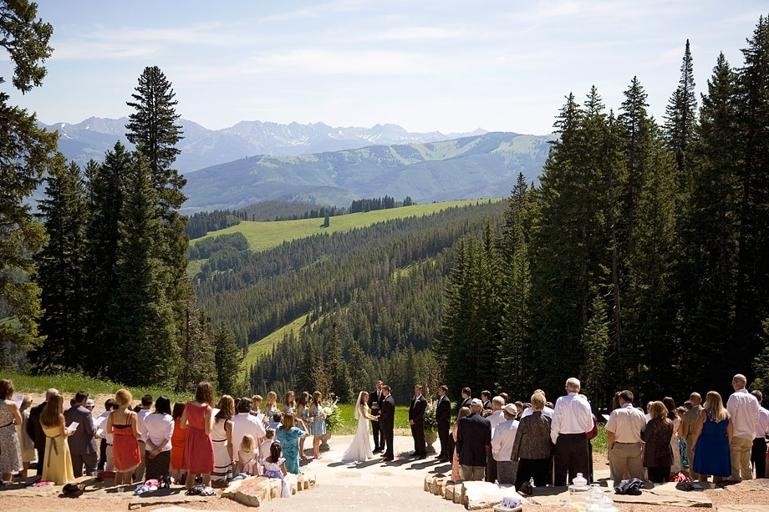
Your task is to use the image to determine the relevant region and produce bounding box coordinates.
[341,391,381,465]
[409,374,769,494]
[1,381,327,487]
[376,385,395,461]
[367,380,386,454]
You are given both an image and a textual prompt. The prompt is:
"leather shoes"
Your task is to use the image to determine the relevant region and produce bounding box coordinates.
[435,454,451,462]
[380,452,392,461]
[410,450,425,459]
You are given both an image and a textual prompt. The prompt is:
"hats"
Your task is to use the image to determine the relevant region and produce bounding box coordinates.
[501,403,517,415]
[471,398,483,407]
[87,399,95,406]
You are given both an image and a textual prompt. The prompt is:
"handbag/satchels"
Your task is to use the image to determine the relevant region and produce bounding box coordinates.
[615,478,642,495]
